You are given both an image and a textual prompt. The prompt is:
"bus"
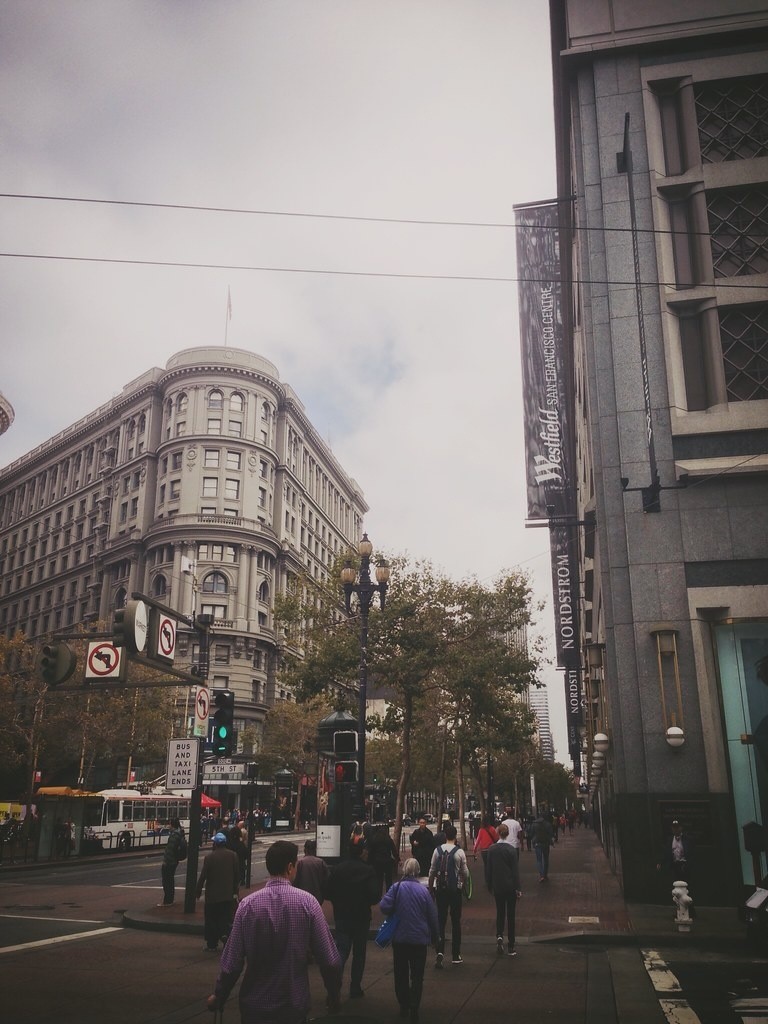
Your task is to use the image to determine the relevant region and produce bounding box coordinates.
[70,790,191,852]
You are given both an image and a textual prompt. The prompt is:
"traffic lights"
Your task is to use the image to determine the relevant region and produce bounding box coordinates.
[334,730,358,754]
[112,598,147,653]
[213,691,235,757]
[373,773,379,785]
[37,640,76,686]
[334,760,358,782]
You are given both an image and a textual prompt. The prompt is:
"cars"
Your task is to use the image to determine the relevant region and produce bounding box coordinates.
[388,814,411,827]
[423,813,450,824]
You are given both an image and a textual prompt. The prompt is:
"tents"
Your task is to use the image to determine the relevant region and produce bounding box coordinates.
[201,793,222,818]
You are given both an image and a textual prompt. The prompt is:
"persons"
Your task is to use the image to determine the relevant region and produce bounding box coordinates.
[198,808,278,845]
[368,824,402,902]
[410,818,435,877]
[535,808,590,836]
[0,809,76,866]
[328,843,375,1000]
[347,812,372,858]
[527,815,555,883]
[291,840,330,920]
[656,818,697,919]
[469,811,558,852]
[380,857,441,1024]
[485,824,523,956]
[209,841,340,1024]
[213,818,250,887]
[429,826,471,969]
[157,818,186,907]
[196,833,239,952]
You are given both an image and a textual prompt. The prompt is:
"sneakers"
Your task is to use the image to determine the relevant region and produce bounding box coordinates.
[452,954,464,963]
[435,952,443,968]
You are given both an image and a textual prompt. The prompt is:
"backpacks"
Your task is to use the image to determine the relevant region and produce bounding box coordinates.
[436,845,463,893]
[172,829,188,861]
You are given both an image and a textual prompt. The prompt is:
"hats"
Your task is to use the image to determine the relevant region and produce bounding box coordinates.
[211,833,226,843]
[672,820,679,826]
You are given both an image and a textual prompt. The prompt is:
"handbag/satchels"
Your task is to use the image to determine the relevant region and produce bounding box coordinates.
[374,915,399,950]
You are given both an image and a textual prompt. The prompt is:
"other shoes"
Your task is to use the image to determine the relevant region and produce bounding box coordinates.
[497,937,504,954]
[202,946,216,952]
[350,983,364,997]
[539,876,544,882]
[157,903,172,907]
[508,949,516,956]
[222,936,228,950]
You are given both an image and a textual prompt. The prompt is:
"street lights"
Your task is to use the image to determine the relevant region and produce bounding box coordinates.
[341,530,391,821]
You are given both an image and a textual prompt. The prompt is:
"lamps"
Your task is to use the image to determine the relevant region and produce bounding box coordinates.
[648,624,685,746]
[583,642,608,800]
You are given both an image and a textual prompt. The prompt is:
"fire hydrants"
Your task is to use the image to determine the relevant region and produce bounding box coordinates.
[305,820,309,830]
[671,881,694,932]
